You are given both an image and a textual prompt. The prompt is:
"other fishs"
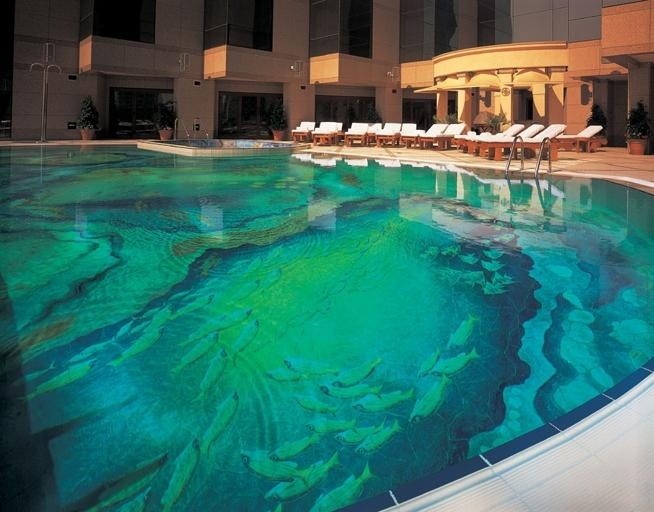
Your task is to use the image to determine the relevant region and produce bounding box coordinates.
[8,235,514,511]
[228,176,309,190]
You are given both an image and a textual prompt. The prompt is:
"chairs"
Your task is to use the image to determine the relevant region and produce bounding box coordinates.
[555,124,603,153]
[291,122,567,161]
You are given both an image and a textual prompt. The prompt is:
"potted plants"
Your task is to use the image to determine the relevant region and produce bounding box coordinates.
[586,103,607,153]
[153,101,176,140]
[270,104,288,141]
[625,101,651,155]
[77,95,102,140]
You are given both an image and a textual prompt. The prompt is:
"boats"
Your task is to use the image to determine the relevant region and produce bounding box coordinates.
[117,121,172,140]
